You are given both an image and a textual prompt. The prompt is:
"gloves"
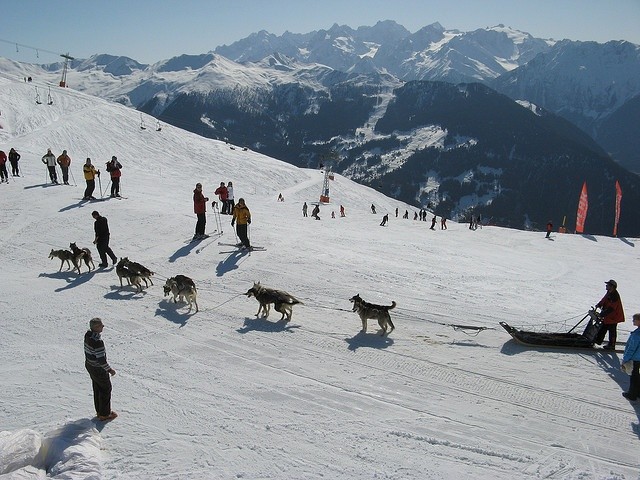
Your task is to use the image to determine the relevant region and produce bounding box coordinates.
[247,220,251,224]
[593,312,600,317]
[231,221,235,227]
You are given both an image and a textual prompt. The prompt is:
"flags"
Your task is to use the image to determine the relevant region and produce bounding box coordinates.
[575,180,589,234]
[613,179,623,235]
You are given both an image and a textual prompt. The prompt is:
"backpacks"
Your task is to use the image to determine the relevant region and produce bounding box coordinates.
[105,161,119,175]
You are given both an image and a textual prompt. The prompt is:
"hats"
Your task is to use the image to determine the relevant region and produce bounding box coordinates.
[47,148,51,151]
[605,280,617,288]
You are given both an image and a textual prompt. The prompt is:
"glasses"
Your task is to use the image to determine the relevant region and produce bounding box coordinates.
[606,285,608,287]
[197,187,201,189]
[100,325,104,327]
[633,320,639,322]
[93,215,96,218]
[240,203,244,204]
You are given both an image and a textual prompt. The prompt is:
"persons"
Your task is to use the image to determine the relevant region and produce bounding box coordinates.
[226,182,235,215]
[313,205,321,220]
[562,226,566,233]
[371,204,376,214]
[340,205,345,217]
[593,279,625,350]
[57,150,71,185]
[83,158,100,200]
[469,214,474,229]
[279,193,282,199]
[441,215,447,230]
[215,182,229,214]
[419,209,422,220]
[106,156,123,198]
[0,151,9,182]
[545,220,553,238]
[405,210,409,219]
[8,148,21,177]
[231,198,251,251]
[302,202,308,217]
[84,318,118,421]
[331,211,336,218]
[558,226,562,233]
[396,208,398,217]
[422,210,427,221]
[92,211,117,268]
[193,183,209,239]
[413,212,418,220]
[430,215,437,229]
[620,313,640,401]
[42,148,59,184]
[312,205,320,216]
[380,214,388,226]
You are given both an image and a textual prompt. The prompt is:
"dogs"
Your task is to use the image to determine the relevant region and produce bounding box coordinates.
[352,301,389,337]
[122,257,155,289]
[116,257,149,294]
[163,281,199,312]
[166,275,197,302]
[69,241,95,273]
[244,287,299,321]
[48,249,85,275]
[252,281,306,316]
[349,293,396,330]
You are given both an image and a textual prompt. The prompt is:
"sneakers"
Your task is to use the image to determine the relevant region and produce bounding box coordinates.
[115,193,120,197]
[114,257,118,264]
[200,234,207,239]
[194,233,202,239]
[100,263,109,267]
[244,246,253,252]
[97,411,115,418]
[603,345,616,350]
[99,414,117,422]
[237,241,245,248]
[110,194,116,198]
[593,340,602,345]
[623,392,639,401]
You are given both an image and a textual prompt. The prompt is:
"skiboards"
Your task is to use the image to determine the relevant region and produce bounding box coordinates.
[107,194,128,200]
[218,242,267,254]
[184,229,223,245]
